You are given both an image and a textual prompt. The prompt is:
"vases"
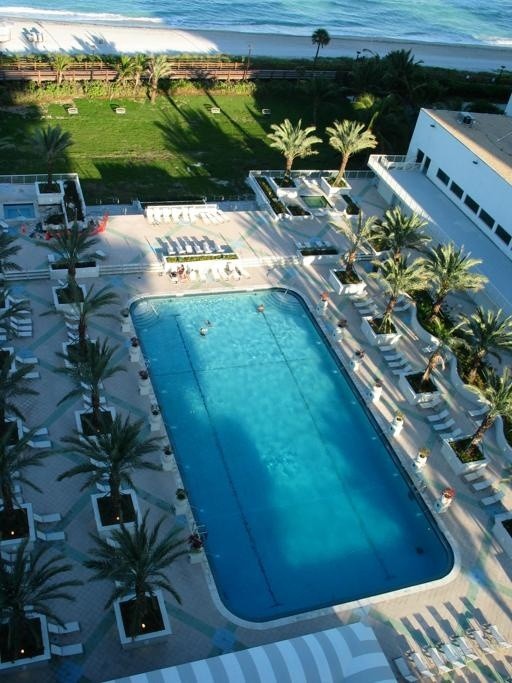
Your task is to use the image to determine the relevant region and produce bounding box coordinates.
[48,260,99,280]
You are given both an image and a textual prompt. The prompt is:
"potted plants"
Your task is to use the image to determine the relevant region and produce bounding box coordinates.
[320,119,378,196]
[0,533,84,674]
[30,123,76,205]
[440,366,512,476]
[361,222,393,257]
[0,419,58,550]
[266,118,323,197]
[398,316,467,405]
[81,506,200,651]
[0,223,23,324]
[0,286,16,379]
[34,221,102,312]
[62,281,123,373]
[55,410,169,538]
[328,208,382,295]
[0,353,41,453]
[361,251,433,346]
[55,335,130,447]
